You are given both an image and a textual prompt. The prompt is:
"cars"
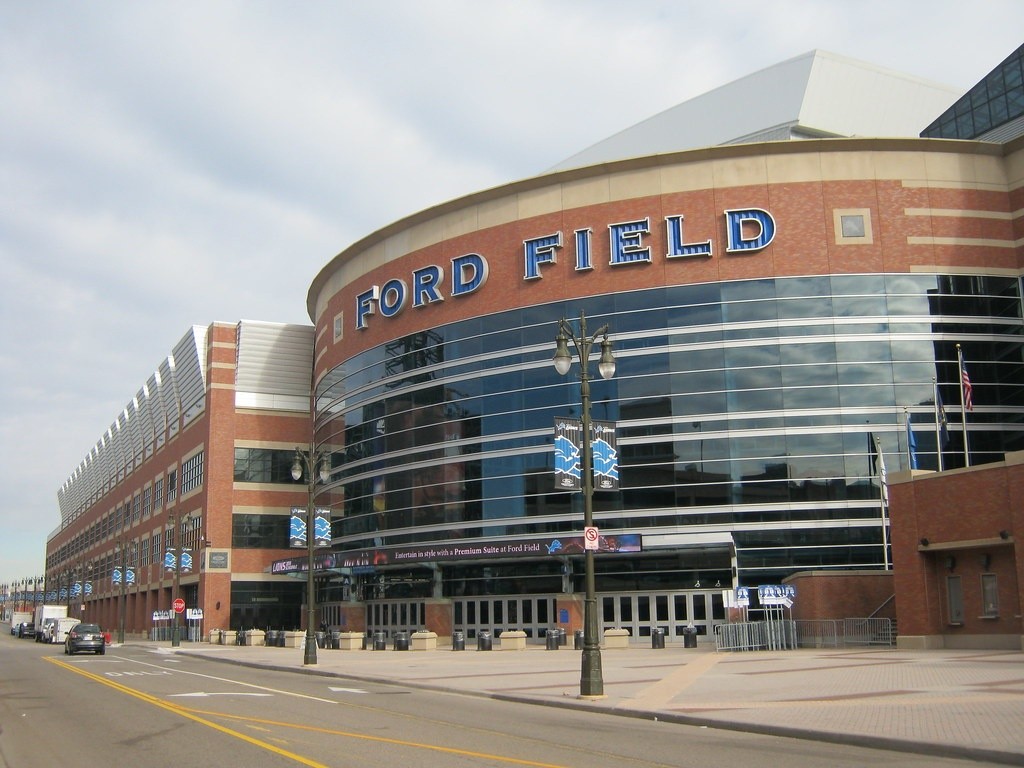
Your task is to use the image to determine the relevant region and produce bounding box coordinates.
[64,623,105,655]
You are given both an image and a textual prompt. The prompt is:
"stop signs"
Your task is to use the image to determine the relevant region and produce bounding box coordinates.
[172,598,186,614]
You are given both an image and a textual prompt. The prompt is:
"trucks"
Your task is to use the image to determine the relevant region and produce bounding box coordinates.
[10,603,82,644]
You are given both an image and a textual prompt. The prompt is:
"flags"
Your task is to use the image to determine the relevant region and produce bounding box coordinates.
[878,447,888,503]
[959,349,974,410]
[936,386,950,446]
[867,430,877,475]
[907,420,917,469]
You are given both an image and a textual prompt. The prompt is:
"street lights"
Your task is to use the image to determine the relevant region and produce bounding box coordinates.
[114,536,137,646]
[290,445,331,667]
[167,507,194,648]
[552,307,617,700]
[76,555,94,623]
